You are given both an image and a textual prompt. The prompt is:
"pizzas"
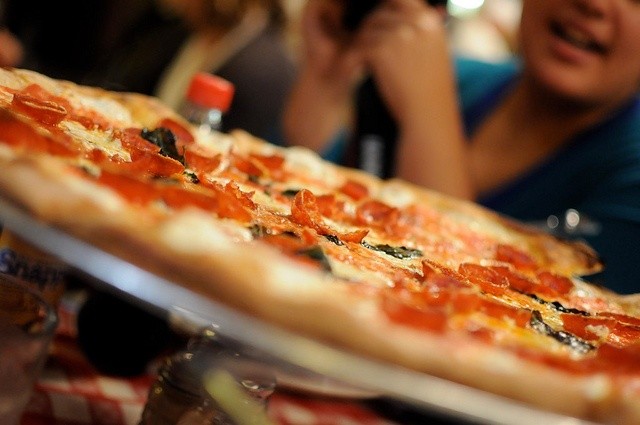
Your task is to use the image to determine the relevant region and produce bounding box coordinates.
[1,67,639,423]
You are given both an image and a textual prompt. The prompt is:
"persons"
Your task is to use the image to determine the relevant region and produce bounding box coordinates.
[1,0,293,143]
[279,0,639,198]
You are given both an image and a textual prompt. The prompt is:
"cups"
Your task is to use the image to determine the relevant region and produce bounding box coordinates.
[0,273,60,424]
